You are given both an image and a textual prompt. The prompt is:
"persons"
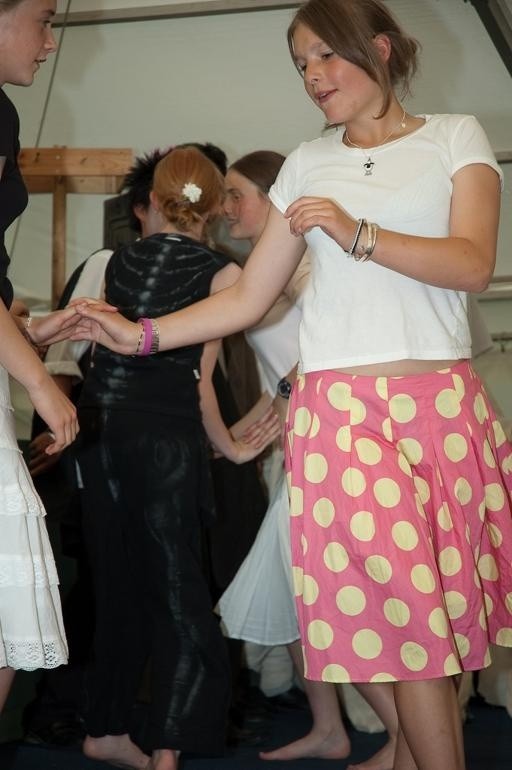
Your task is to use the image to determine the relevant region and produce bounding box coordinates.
[1,0,122,770]
[61,0,512,768]
[2,139,497,770]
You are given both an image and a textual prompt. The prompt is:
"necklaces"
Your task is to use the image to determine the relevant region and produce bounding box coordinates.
[344,108,408,178]
